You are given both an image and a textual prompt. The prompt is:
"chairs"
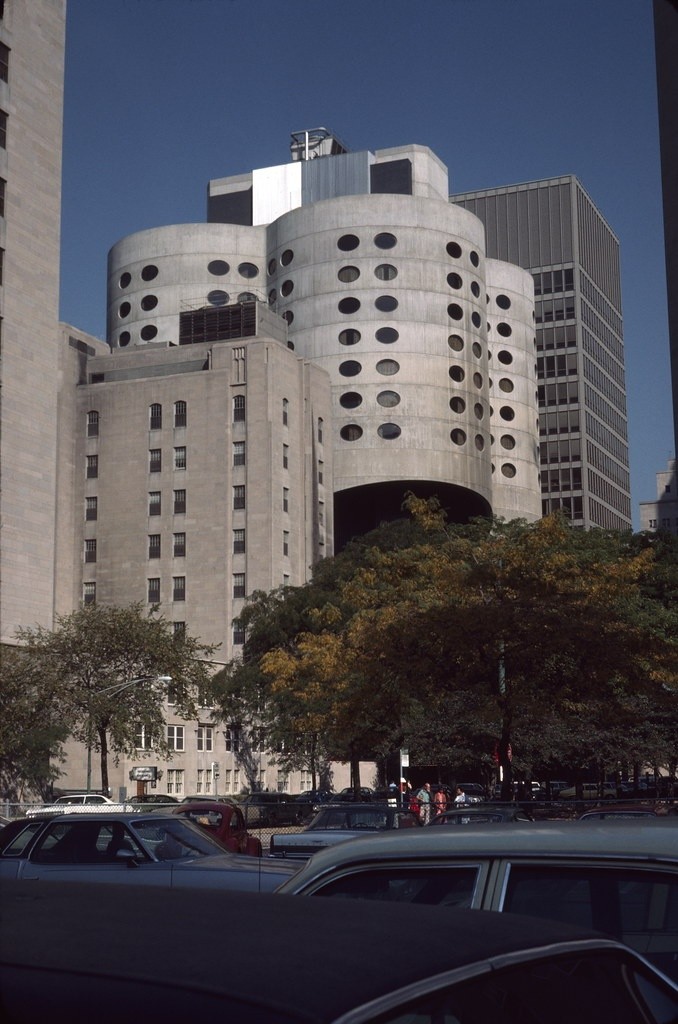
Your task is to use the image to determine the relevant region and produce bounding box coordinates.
[106,838,137,863]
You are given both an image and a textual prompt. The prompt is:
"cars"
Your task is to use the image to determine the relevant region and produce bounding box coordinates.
[170,802,264,859]
[26,795,136,819]
[456,781,559,805]
[425,808,535,824]
[179,796,261,828]
[294,790,336,820]
[269,804,423,860]
[122,795,179,815]
[579,807,658,819]
[331,785,452,809]
[559,783,617,801]
[270,815,677,993]
[0,813,313,895]
[594,775,678,800]
[239,793,297,829]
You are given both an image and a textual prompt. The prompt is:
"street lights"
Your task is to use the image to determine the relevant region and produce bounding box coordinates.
[83,676,174,798]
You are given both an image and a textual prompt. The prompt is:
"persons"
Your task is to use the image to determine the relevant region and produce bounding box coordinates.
[454,787,465,824]
[416,782,435,827]
[434,784,452,825]
[501,778,533,813]
